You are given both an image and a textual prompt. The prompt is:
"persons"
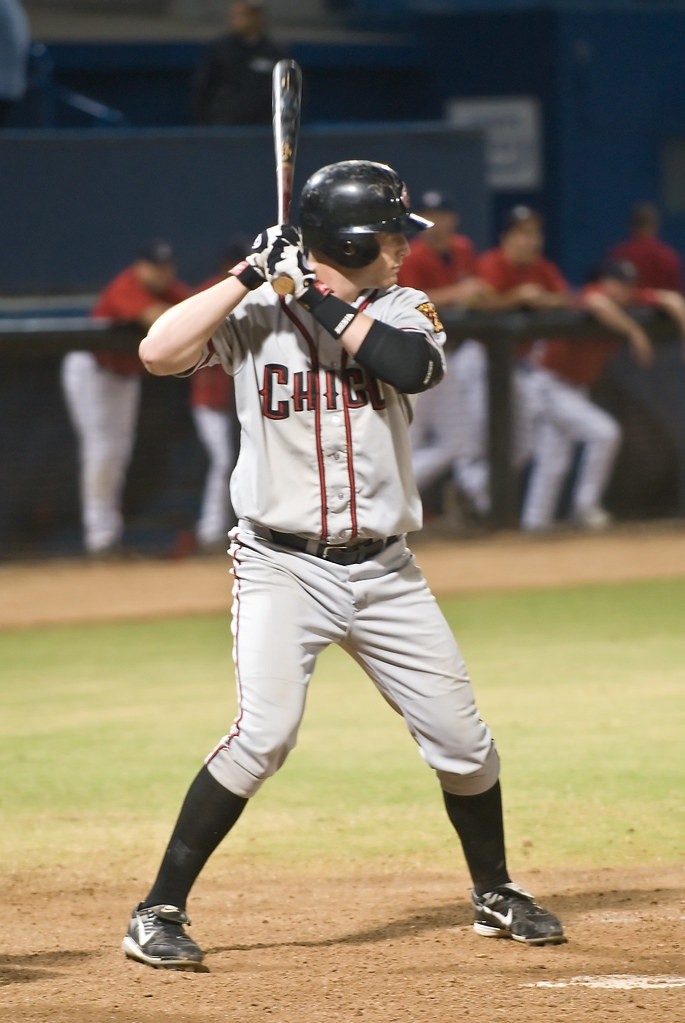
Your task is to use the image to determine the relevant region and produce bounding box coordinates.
[58,239,685,552]
[197,1,274,124]
[0,0,32,129]
[121,160,564,967]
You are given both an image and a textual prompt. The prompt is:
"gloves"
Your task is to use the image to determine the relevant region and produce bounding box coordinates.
[228,223,334,310]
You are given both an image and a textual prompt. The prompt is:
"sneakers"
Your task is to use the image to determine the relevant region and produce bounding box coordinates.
[471,882,569,944]
[122,901,202,966]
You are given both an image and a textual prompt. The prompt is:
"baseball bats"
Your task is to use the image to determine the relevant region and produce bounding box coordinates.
[269,56,305,298]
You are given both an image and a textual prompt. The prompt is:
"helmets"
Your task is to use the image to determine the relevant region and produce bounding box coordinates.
[302,159,436,269]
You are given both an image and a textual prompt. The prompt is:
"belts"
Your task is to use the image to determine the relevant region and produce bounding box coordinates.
[269,528,399,566]
